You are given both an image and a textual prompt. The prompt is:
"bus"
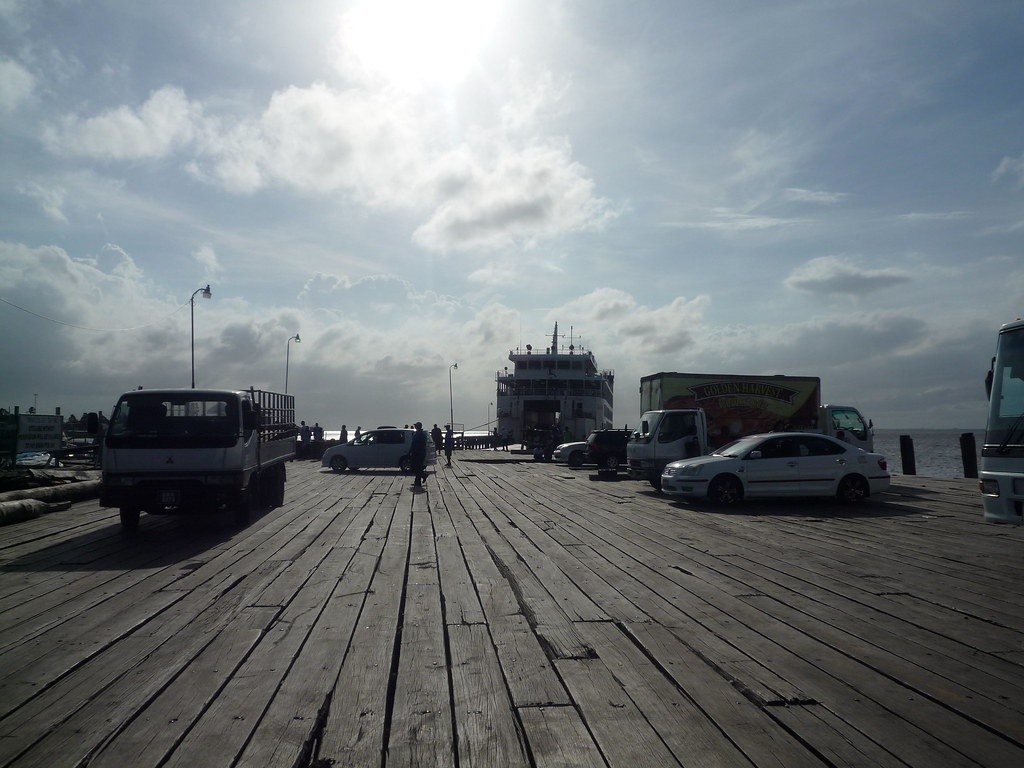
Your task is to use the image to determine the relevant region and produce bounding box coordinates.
[976,316,1024,530]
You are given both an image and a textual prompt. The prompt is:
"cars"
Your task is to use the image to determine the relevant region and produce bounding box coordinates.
[660,429,892,517]
[551,441,595,468]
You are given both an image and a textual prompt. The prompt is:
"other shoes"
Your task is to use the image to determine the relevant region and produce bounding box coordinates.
[445,464,450,466]
[447,463,451,465]
[422,472,429,483]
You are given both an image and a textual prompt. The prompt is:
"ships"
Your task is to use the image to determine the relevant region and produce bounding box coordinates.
[492,320,615,443]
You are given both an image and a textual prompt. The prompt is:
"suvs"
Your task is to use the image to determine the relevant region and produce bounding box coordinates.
[582,423,635,470]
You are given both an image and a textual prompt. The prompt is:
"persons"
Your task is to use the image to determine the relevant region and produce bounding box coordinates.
[298,426,312,461]
[444,425,453,466]
[489,424,573,452]
[408,422,429,488]
[313,423,324,460]
[354,426,361,437]
[300,421,309,440]
[431,424,441,455]
[340,424,348,444]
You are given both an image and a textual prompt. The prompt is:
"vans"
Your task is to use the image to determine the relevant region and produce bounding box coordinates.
[321,425,437,474]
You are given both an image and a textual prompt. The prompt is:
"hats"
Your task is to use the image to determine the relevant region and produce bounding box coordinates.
[414,422,422,428]
[444,425,450,427]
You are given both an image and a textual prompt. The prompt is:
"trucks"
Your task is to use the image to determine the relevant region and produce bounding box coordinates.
[625,406,719,491]
[641,371,875,451]
[98,383,300,530]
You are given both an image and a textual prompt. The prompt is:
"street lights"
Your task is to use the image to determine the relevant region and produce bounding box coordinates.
[448,362,458,430]
[284,333,301,394]
[488,402,494,435]
[191,285,212,388]
